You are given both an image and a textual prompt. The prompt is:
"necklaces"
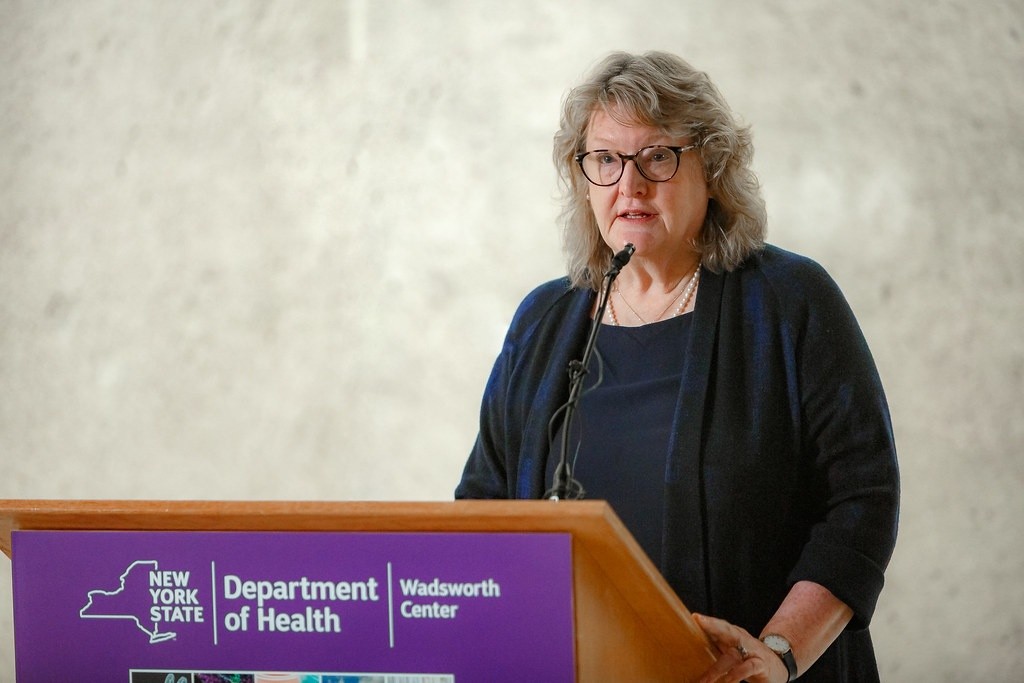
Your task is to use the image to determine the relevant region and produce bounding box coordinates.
[604,259,701,326]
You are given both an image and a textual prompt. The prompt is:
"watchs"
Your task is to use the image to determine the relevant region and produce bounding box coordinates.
[759,634,798,683]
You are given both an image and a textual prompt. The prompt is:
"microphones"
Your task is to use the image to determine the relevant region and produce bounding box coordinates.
[549,242,636,500]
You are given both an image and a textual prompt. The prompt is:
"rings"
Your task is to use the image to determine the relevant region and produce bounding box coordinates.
[735,645,748,659]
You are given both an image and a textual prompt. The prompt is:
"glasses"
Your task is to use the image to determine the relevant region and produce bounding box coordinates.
[574,140,703,187]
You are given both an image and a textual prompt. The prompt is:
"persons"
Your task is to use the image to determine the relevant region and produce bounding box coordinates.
[454,53,900,683]
